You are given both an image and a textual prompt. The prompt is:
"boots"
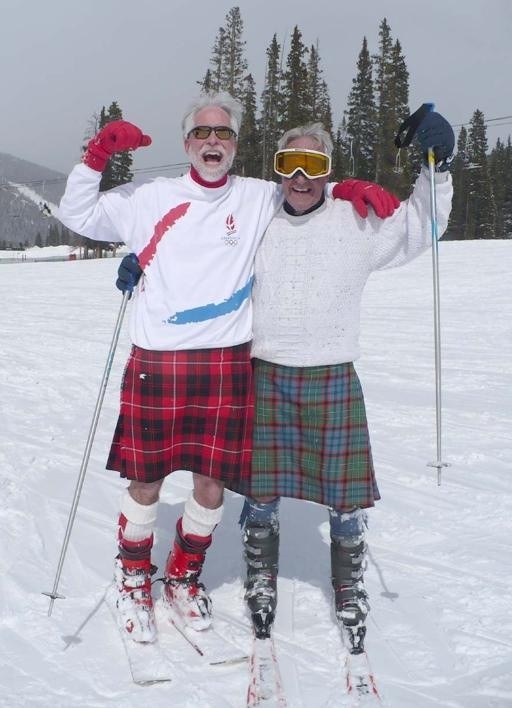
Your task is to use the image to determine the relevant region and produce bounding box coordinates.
[163,517,213,630]
[329,509,370,656]
[242,523,278,638]
[113,513,158,643]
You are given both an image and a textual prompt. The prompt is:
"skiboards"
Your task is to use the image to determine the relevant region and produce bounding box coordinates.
[246,638,385,708]
[105,586,249,684]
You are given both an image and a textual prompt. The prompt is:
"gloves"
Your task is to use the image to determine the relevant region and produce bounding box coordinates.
[85,120,151,171]
[115,254,143,300]
[416,112,454,168]
[333,179,399,218]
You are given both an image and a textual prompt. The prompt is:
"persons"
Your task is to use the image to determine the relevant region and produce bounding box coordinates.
[238,109,465,623]
[54,91,402,643]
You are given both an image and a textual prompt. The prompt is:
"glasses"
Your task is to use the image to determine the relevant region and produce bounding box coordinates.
[187,125,238,140]
[273,149,333,180]
